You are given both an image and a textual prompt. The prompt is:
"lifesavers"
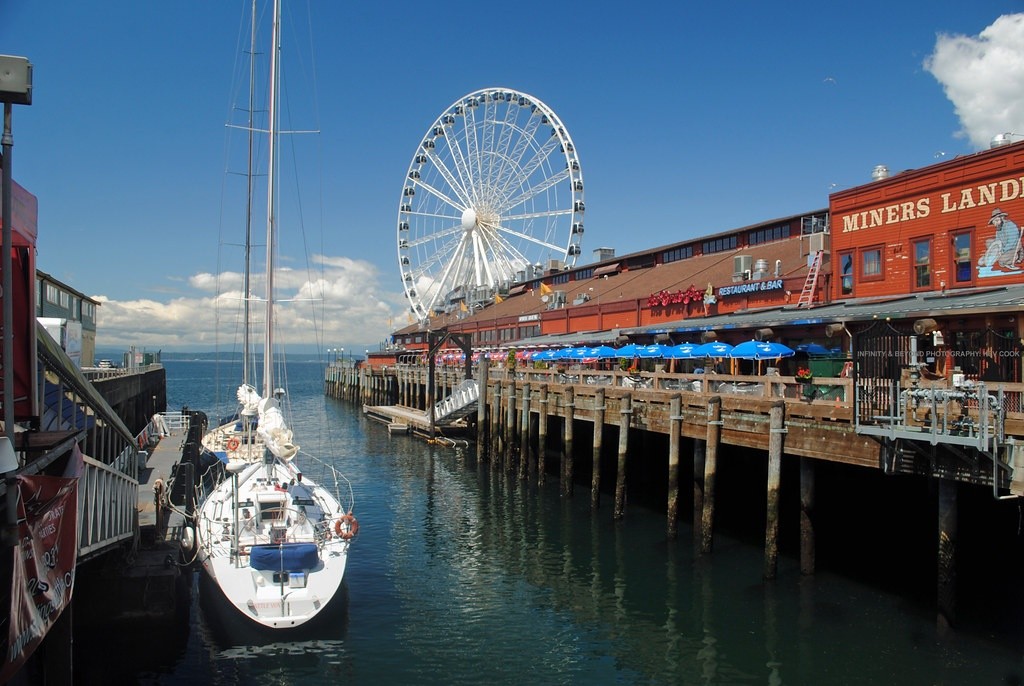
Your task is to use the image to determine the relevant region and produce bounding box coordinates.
[138,435,143,449]
[143,432,149,444]
[226,437,240,451]
[334,514,359,539]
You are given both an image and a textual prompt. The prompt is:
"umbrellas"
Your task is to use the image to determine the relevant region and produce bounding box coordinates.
[421,340,795,383]
[794,340,841,357]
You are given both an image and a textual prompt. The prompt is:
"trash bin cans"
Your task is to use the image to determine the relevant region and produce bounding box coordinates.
[138,451,148,470]
[807,352,847,402]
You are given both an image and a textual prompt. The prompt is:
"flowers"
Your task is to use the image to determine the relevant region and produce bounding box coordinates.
[556,364,565,370]
[627,366,640,375]
[795,365,813,379]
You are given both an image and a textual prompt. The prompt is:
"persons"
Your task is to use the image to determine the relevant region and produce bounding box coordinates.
[691,363,704,382]
[714,360,725,374]
[393,340,398,349]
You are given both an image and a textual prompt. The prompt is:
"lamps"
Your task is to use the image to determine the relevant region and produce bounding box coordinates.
[825,183,853,191]
[932,151,957,159]
[1003,132,1024,142]
[878,168,904,173]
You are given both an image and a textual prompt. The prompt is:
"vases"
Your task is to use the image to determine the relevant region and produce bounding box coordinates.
[795,377,812,384]
[629,373,639,376]
[557,369,565,373]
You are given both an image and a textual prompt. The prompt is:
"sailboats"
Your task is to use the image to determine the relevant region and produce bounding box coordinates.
[151,0,362,631]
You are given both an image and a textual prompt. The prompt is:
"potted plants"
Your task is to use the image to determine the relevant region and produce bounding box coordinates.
[507,349,516,369]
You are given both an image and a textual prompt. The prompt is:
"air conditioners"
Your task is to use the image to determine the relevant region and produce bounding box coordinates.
[733,254,753,274]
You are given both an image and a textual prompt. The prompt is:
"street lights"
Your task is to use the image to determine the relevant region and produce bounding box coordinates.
[333,348,336,367]
[327,349,330,368]
[341,347,344,368]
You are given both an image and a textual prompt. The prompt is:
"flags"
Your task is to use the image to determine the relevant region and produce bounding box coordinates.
[541,282,551,296]
[496,294,503,304]
[429,308,435,318]
[460,301,467,312]
[409,315,412,323]
